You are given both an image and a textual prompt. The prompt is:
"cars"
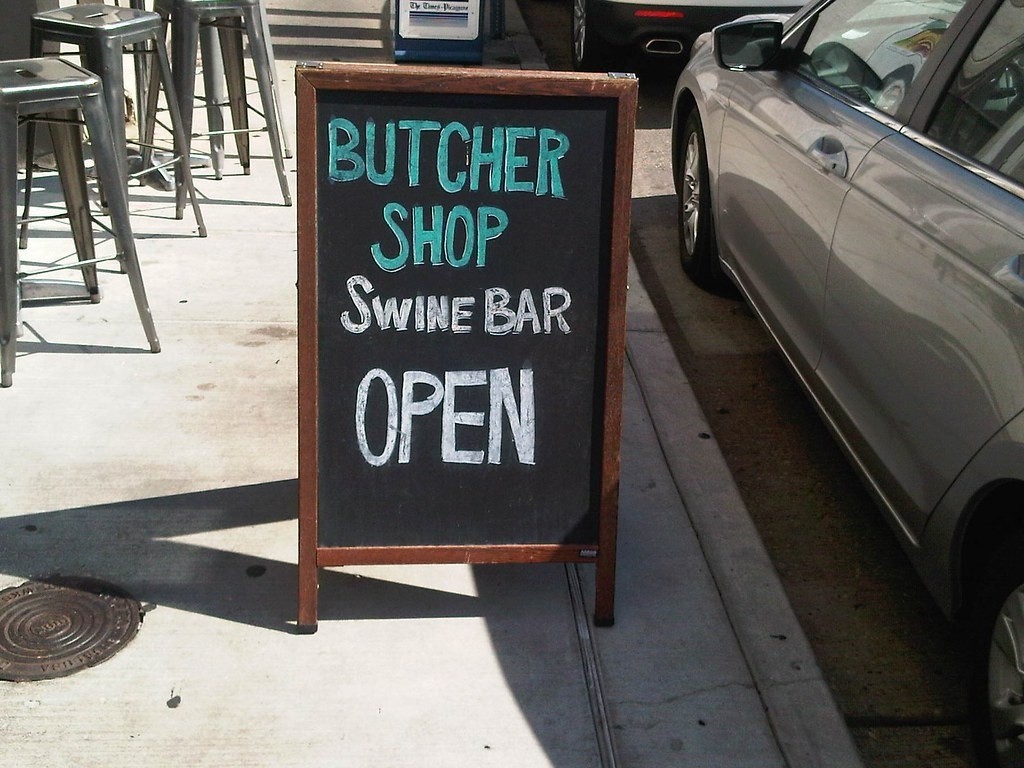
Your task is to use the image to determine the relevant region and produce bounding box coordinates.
[670,0,1024,768]
[571,0,805,73]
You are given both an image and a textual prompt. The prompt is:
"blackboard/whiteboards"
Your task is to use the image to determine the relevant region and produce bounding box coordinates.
[295,61,639,567]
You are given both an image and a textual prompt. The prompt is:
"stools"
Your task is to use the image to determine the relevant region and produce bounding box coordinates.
[0,54,161,388]
[17,4,207,273]
[139,0,292,223]
